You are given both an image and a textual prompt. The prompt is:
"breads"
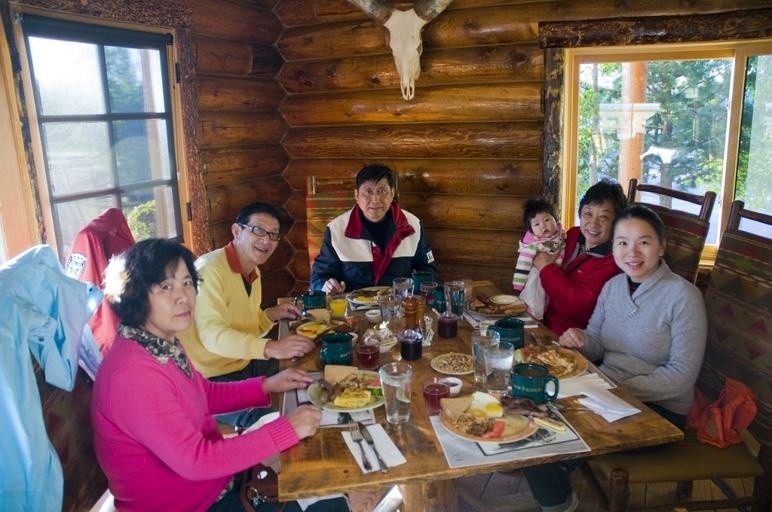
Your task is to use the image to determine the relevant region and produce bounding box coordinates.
[306,309,330,325]
[440,396,475,418]
[325,365,358,385]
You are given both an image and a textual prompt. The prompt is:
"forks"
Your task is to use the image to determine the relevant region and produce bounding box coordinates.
[348,420,372,471]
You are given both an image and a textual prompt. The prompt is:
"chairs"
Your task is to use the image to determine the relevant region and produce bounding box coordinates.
[628,180,715,284]
[306,171,405,274]
[578,201,772,512]
[0,245,110,512]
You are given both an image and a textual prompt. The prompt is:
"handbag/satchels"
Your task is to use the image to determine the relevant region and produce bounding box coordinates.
[240,462,288,512]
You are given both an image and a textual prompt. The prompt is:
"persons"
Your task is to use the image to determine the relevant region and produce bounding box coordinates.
[310,162,438,296]
[532,179,627,335]
[511,199,568,321]
[90,238,349,509]
[520,206,708,512]
[174,200,316,432]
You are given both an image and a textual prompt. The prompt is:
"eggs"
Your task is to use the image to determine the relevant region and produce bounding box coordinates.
[471,391,504,419]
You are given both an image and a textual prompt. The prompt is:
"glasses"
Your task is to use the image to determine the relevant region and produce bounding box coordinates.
[239,221,282,241]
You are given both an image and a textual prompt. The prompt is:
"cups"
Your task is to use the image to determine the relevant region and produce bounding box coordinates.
[365,310,381,324]
[350,333,358,347]
[511,363,559,404]
[319,333,353,368]
[328,290,349,318]
[355,338,380,370]
[379,362,415,423]
[470,317,526,396]
[440,378,463,395]
[422,377,451,415]
[380,278,466,360]
[293,291,326,315]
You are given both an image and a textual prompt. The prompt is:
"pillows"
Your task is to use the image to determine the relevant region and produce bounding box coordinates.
[78,208,136,354]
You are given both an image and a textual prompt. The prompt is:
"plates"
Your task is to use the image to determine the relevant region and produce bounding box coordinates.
[430,353,474,375]
[510,345,587,381]
[489,295,518,304]
[439,409,542,442]
[346,286,392,304]
[290,320,348,338]
[306,370,382,412]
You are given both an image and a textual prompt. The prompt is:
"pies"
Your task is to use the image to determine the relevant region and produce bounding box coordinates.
[334,388,372,408]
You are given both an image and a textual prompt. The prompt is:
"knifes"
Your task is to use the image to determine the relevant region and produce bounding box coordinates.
[358,421,389,474]
[529,330,547,354]
[318,378,333,392]
[291,328,330,361]
